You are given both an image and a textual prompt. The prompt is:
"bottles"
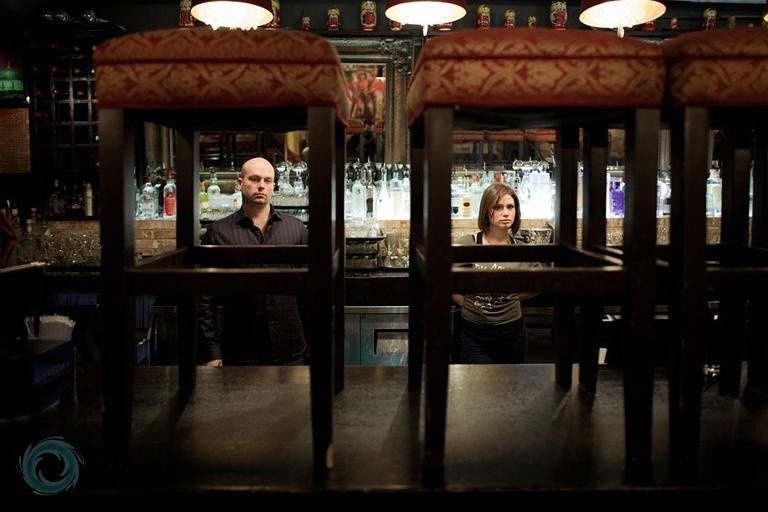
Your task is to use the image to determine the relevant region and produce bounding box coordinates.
[137,167,243,219]
[357,167,378,221]
[10,208,44,263]
[611,183,625,219]
[48,180,96,218]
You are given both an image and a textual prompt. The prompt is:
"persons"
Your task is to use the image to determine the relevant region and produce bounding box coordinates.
[452,183,548,364]
[199,156,315,366]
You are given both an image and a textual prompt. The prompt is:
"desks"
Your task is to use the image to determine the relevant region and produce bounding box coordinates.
[72,360,767,510]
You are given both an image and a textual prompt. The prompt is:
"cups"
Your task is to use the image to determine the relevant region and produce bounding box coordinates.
[277,162,522,196]
